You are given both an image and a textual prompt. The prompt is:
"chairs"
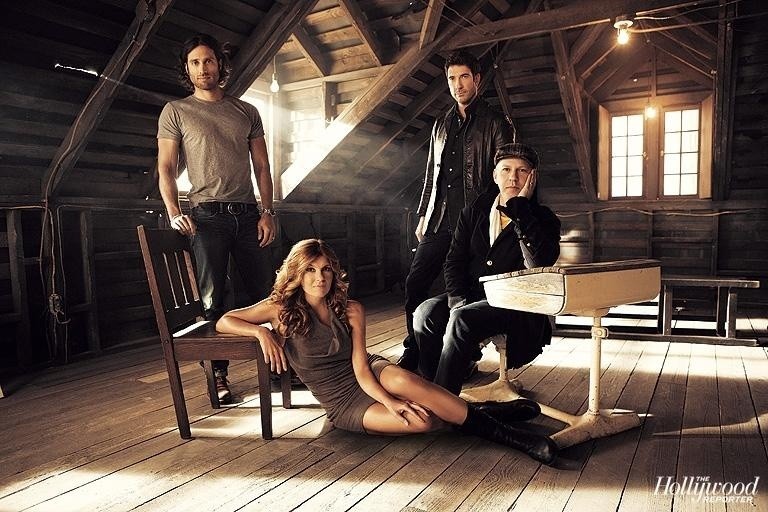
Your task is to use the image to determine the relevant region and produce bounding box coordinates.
[138,221,294,441]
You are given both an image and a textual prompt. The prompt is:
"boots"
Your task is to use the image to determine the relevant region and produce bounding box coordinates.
[468,399,542,424]
[462,401,560,466]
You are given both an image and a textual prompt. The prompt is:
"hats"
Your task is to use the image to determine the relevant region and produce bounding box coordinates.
[493,143,540,168]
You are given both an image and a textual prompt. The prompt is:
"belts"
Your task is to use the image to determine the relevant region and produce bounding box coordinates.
[191,201,259,213]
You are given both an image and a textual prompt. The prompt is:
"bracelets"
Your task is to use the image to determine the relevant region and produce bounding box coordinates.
[170,213,182,222]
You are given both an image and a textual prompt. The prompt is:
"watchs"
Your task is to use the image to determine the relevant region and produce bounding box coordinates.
[260,207,276,216]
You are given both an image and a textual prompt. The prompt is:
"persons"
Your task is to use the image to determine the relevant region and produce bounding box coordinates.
[398,52,514,372]
[157,37,303,407]
[412,141,562,397]
[215,238,557,464]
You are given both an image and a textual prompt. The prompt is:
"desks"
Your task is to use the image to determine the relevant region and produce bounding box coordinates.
[452,258,665,448]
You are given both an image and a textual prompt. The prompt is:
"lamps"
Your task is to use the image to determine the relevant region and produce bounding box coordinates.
[614,15,634,46]
[269,74,279,93]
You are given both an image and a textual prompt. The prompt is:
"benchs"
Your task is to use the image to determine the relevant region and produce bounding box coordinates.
[554,207,760,347]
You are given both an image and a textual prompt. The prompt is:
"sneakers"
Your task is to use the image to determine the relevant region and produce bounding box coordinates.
[271,377,304,388]
[206,376,233,405]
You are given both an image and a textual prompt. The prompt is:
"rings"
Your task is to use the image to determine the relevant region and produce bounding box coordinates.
[530,183,533,184]
